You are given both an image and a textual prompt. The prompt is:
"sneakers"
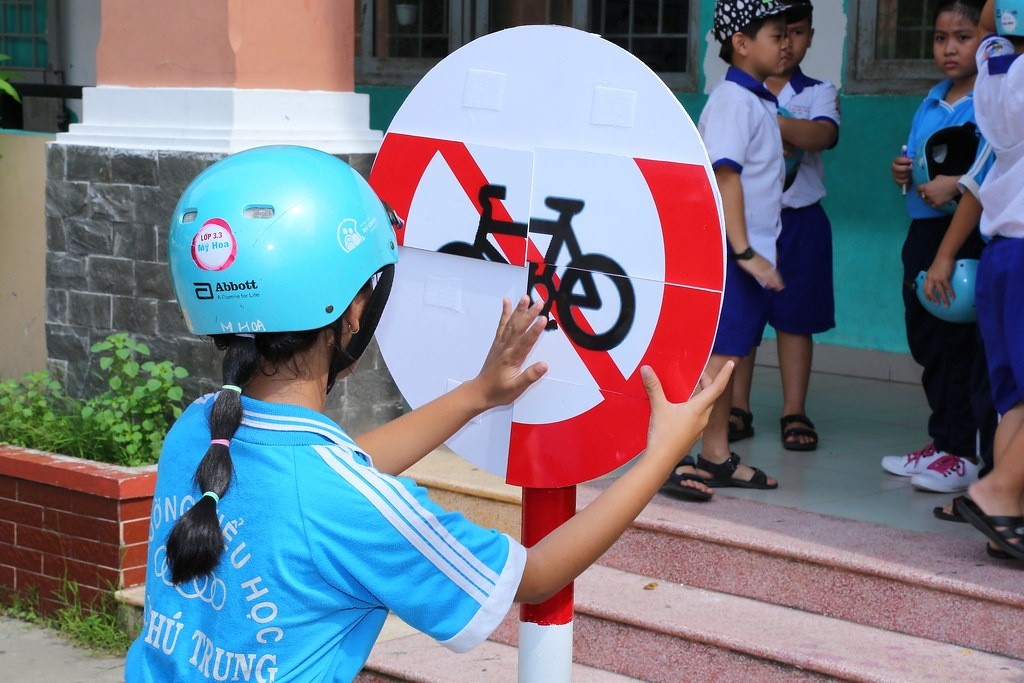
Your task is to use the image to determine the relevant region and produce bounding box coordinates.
[882,443,983,493]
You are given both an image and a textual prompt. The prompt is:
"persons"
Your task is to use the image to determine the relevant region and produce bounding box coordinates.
[881,1,985,493]
[959,0,1024,559]
[655,0,802,501]
[923,132,998,521]
[729,0,843,447]
[120,146,735,683]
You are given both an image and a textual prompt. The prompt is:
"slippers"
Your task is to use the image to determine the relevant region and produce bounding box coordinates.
[954,496,1024,558]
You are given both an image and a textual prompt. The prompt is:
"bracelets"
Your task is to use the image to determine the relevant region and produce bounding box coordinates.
[736,247,754,260]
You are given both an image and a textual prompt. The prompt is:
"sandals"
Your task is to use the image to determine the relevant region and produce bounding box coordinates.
[934,503,957,522]
[728,406,755,443]
[780,413,819,451]
[697,451,780,490]
[658,456,714,501]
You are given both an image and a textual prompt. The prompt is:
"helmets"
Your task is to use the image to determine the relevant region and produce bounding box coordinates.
[993,0,1023,38]
[167,144,400,335]
[913,259,982,323]
[776,106,804,192]
[911,121,982,215]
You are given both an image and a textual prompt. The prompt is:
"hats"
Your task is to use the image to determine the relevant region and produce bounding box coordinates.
[776,0,814,24]
[714,0,793,45]
